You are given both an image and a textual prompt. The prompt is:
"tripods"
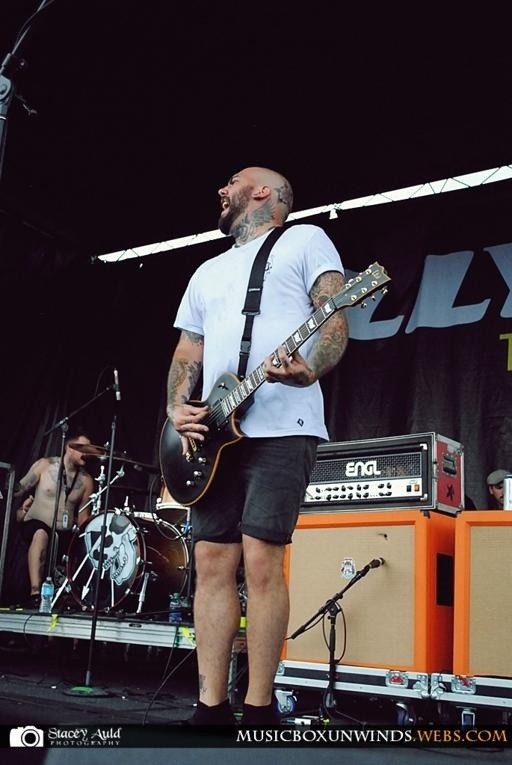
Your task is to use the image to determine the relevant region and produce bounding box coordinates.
[9,385,112,612]
[281,569,369,728]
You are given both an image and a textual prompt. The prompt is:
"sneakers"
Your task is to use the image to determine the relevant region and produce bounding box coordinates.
[30,594,41,608]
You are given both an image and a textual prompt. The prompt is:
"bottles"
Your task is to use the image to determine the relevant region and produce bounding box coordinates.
[39,576,54,613]
[169,592,182,621]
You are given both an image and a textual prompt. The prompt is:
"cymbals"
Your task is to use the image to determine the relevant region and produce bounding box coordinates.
[68,440,126,456]
[81,452,158,476]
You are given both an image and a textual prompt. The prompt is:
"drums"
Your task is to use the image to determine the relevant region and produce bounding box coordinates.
[68,511,190,613]
[154,503,192,545]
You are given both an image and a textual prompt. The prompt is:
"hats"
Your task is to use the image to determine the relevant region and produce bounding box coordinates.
[487,470,506,484]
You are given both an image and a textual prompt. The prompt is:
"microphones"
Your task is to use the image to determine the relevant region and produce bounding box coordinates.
[113,366,122,400]
[365,558,384,569]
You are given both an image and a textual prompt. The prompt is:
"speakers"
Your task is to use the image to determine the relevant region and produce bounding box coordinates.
[279,509,456,674]
[453,510,512,678]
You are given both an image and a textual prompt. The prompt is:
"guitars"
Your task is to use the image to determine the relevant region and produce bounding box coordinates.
[159,262,392,507]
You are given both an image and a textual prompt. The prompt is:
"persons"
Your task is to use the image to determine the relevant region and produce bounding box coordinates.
[0,433,99,610]
[162,167,352,728]
[486,468,510,510]
[22,487,36,513]
